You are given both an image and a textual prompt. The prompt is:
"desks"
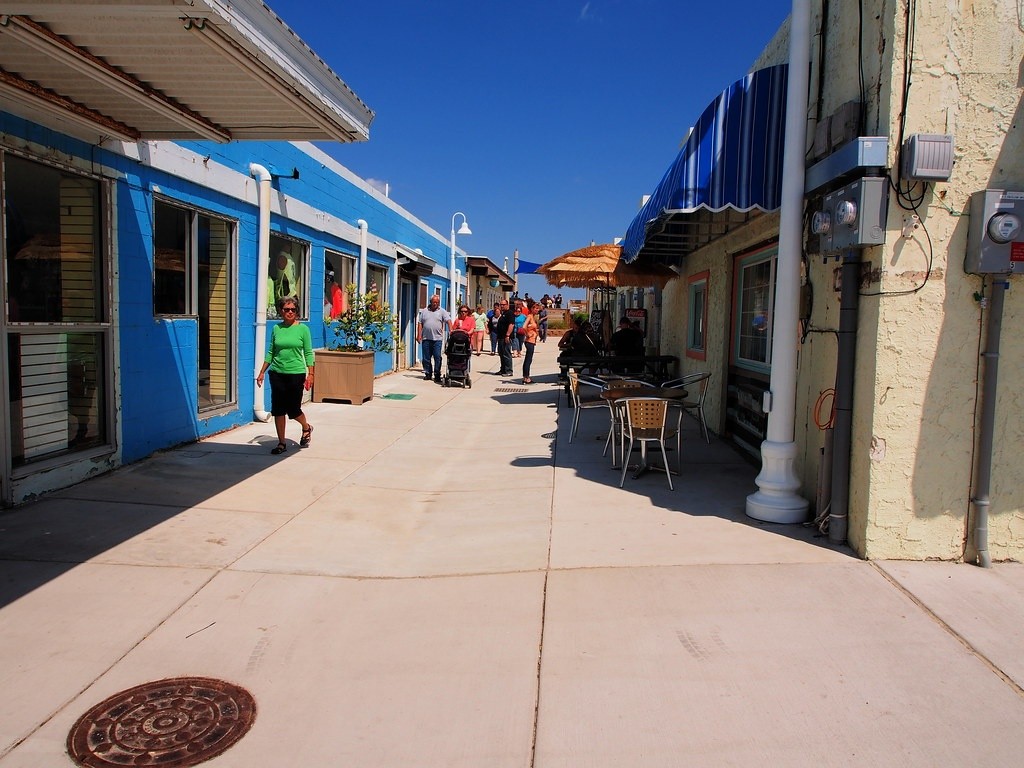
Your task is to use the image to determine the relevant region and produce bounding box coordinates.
[600,387,688,479]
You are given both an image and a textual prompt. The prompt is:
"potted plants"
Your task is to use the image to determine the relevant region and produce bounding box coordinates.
[310,284,407,406]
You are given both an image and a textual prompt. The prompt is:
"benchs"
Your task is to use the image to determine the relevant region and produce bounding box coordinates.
[556,355,673,408]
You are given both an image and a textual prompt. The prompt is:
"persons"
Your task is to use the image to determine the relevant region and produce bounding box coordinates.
[558,315,645,376]
[452,305,476,348]
[416,295,453,383]
[494,299,515,376]
[472,304,489,355]
[275,244,295,300]
[488,292,561,358]
[256,299,314,455]
[522,304,540,384]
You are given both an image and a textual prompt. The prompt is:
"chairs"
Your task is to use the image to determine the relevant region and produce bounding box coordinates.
[566,370,712,490]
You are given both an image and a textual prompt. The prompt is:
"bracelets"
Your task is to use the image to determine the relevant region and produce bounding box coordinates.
[308,373,314,376]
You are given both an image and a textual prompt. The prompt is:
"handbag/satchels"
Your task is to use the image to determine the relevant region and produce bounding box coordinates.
[512,333,520,350]
[518,328,529,334]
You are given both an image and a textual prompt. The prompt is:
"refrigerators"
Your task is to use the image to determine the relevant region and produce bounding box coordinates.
[626,308,647,354]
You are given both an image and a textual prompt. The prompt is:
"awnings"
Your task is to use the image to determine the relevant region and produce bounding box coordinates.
[621,63,788,267]
[0,0,375,145]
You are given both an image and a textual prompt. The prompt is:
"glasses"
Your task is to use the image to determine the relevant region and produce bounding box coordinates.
[460,310,468,313]
[282,308,296,312]
[500,304,507,306]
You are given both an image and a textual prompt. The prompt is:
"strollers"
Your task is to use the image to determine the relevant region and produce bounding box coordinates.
[441,329,472,388]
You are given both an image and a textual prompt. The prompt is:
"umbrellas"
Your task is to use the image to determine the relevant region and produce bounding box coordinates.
[539,242,678,337]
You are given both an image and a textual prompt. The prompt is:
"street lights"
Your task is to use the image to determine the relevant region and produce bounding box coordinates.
[450,211,472,324]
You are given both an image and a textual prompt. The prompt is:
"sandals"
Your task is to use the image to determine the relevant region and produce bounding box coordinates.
[271,443,287,454]
[300,424,313,446]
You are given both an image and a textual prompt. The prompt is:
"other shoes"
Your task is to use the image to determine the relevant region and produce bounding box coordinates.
[434,377,441,383]
[522,380,536,385]
[543,340,545,343]
[477,353,480,356]
[502,372,513,376]
[423,376,432,380]
[491,353,494,355]
[540,338,543,341]
[494,371,503,375]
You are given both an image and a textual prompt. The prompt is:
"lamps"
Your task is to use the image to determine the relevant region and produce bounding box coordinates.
[0,15,356,144]
[274,168,299,180]
